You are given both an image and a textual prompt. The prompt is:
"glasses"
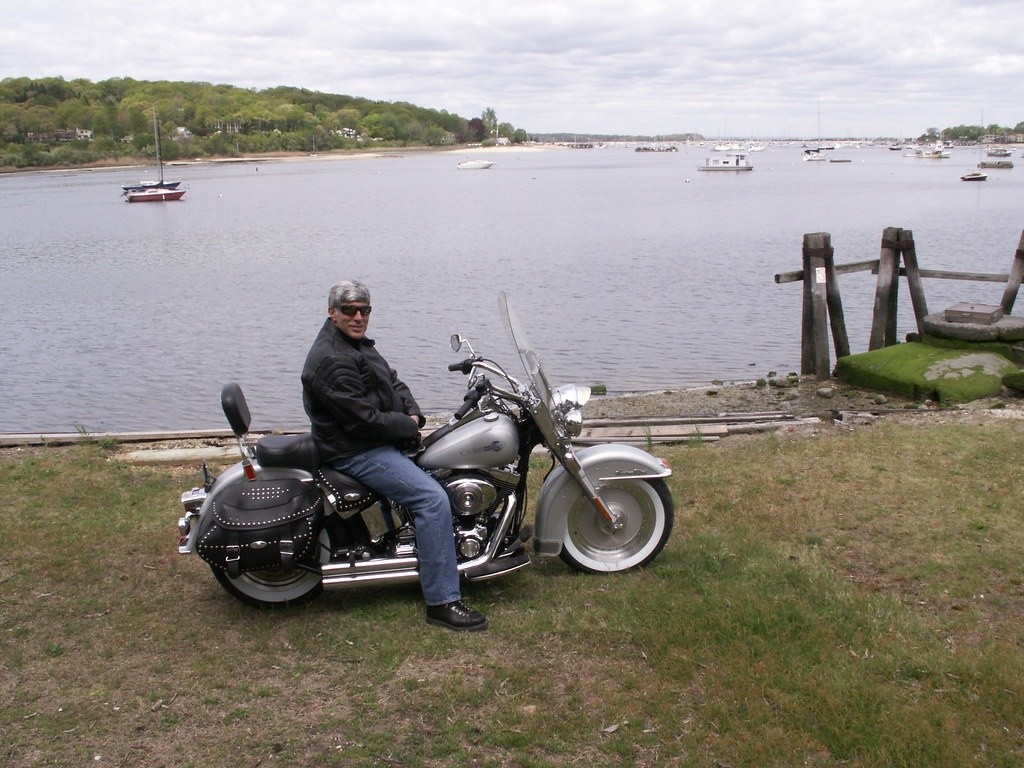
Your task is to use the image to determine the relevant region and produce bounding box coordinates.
[334,304,372,316]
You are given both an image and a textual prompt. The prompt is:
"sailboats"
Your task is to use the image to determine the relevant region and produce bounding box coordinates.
[124,104,187,203]
[567,101,955,162]
[978,109,1015,170]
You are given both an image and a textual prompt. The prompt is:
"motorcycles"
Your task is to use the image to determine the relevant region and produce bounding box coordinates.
[175,290,674,611]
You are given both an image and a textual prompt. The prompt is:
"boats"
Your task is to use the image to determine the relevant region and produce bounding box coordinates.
[699,152,754,172]
[456,160,494,170]
[962,170,989,182]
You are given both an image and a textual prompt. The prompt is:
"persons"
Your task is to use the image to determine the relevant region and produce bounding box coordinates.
[301,278,489,632]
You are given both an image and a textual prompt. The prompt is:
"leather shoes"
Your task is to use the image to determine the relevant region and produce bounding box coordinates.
[425,599,489,631]
